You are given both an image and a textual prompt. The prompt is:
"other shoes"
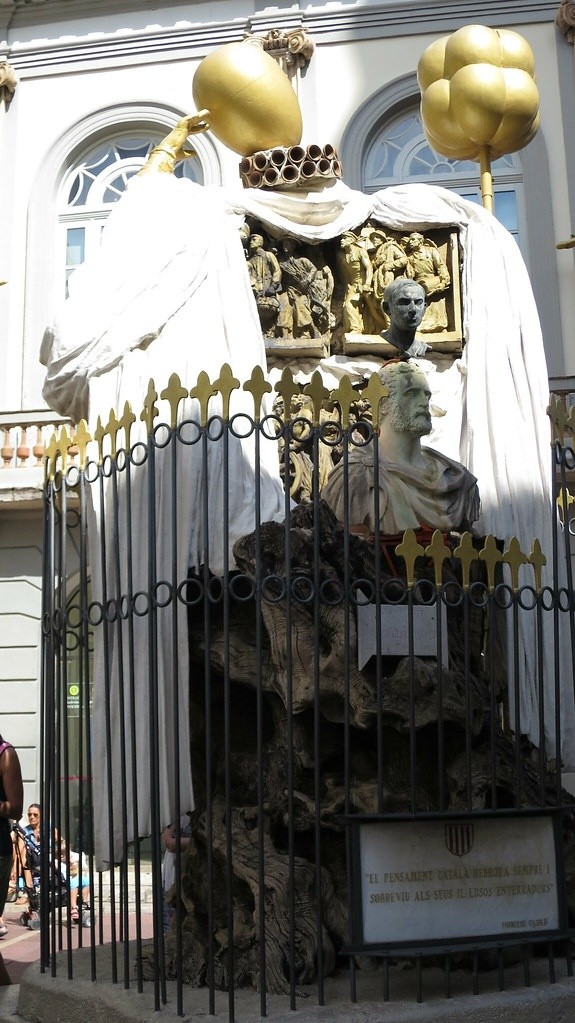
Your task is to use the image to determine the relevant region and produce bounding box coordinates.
[0,923,8,937]
[8,881,16,894]
[72,909,79,919]
[15,896,27,905]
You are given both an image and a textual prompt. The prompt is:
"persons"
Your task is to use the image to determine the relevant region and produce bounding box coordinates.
[319,361,481,533]
[0,734,24,937]
[161,809,191,935]
[270,395,366,504]
[9,804,96,920]
[240,222,450,357]
[39,43,574,874]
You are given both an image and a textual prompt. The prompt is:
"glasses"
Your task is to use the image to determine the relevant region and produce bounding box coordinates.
[28,813,39,817]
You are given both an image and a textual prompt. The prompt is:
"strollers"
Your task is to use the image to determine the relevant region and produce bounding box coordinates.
[7,818,98,931]
